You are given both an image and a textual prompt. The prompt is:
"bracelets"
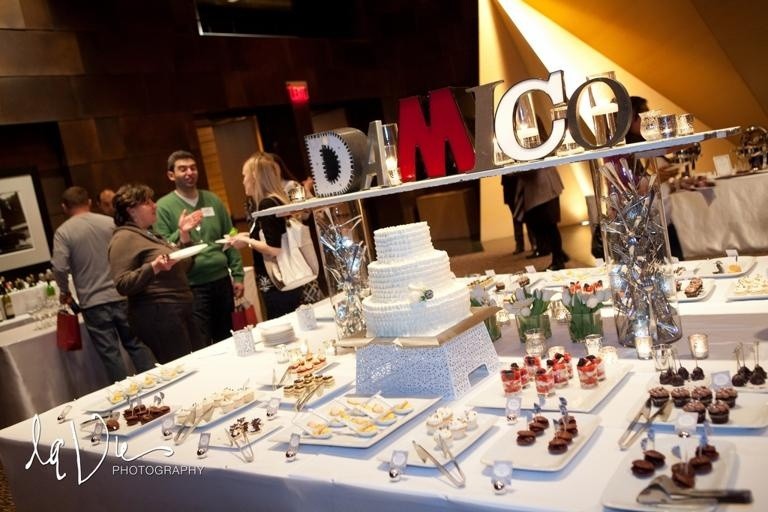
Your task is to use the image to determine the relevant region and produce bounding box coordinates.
[248,238,255,249]
[682,175,689,189]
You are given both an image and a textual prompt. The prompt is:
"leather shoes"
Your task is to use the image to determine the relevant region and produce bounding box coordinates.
[512,247,570,271]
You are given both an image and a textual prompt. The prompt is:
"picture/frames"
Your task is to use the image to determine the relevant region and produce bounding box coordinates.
[0,164,56,281]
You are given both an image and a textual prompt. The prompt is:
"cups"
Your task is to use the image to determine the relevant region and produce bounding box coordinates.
[551,107,582,157]
[637,111,693,141]
[289,186,306,204]
[387,168,404,186]
[379,124,399,186]
[586,72,624,145]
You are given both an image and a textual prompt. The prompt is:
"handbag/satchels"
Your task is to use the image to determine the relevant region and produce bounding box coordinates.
[252,197,319,292]
[56,304,83,353]
[231,295,259,331]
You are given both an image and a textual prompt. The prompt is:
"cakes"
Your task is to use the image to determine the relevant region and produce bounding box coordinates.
[176,386,263,439]
[283,352,336,399]
[501,353,608,455]
[303,396,413,448]
[362,220,471,338]
[426,406,478,450]
[631,388,739,490]
[105,363,189,431]
[676,259,768,299]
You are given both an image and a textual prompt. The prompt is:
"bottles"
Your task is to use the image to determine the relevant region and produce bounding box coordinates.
[0,277,16,318]
[46,281,55,298]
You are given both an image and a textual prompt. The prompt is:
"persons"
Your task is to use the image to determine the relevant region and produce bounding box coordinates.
[151,151,245,345]
[501,173,537,256]
[602,98,680,265]
[300,175,314,221]
[229,151,302,319]
[625,96,696,261]
[269,152,326,303]
[50,186,156,384]
[512,102,561,260]
[107,184,203,365]
[97,188,118,217]
[517,168,570,271]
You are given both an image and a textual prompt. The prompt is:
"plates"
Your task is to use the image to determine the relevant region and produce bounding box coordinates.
[81,255,768,512]
[216,240,236,244]
[167,242,207,260]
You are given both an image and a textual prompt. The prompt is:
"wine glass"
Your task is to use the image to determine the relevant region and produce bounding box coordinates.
[27,300,69,330]
